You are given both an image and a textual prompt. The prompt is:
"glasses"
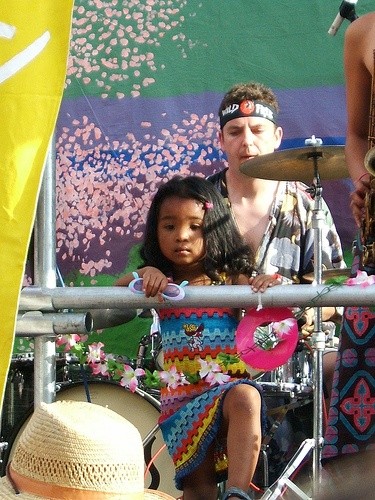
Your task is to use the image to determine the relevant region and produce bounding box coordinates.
[128,271,189,302]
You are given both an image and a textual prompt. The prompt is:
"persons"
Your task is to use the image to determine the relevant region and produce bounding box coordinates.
[112,174,289,500]
[321,10,375,483]
[202,82,349,482]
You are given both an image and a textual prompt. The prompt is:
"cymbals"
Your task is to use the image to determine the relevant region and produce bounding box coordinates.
[239,146,351,181]
[303,268,351,282]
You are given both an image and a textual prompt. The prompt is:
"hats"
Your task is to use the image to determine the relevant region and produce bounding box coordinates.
[0,401,176,500]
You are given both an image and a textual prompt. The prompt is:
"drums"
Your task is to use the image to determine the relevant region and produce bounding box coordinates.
[254,337,314,415]
[1,380,220,500]
[8,354,82,428]
